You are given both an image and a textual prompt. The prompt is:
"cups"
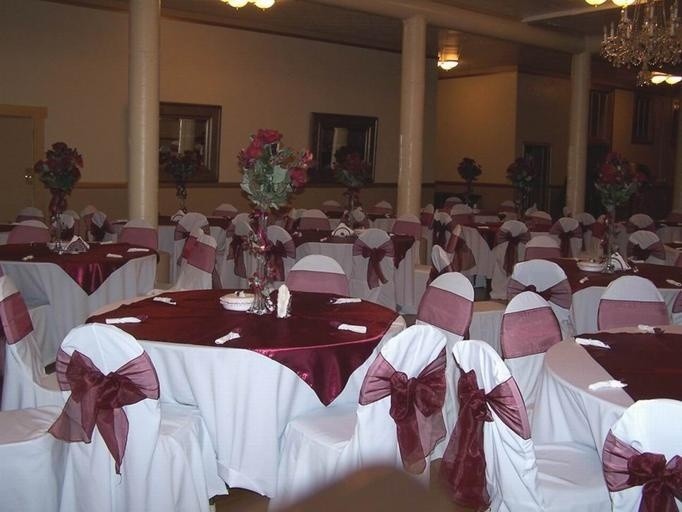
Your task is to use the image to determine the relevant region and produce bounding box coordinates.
[277,293,295,321]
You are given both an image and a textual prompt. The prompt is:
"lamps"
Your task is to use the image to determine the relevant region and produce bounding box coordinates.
[220,0,275,12]
[435,31,460,72]
[585,0,682,89]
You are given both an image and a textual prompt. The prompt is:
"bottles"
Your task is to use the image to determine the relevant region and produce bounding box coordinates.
[53,242,63,256]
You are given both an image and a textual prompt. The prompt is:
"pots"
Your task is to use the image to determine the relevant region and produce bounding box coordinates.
[47,239,70,251]
[576,260,604,272]
[355,226,364,237]
[220,291,256,312]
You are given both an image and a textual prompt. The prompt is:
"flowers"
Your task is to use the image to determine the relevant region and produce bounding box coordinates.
[33,142,84,221]
[454,156,482,194]
[238,128,315,237]
[157,142,207,193]
[591,149,650,221]
[507,153,541,206]
[329,145,375,194]
